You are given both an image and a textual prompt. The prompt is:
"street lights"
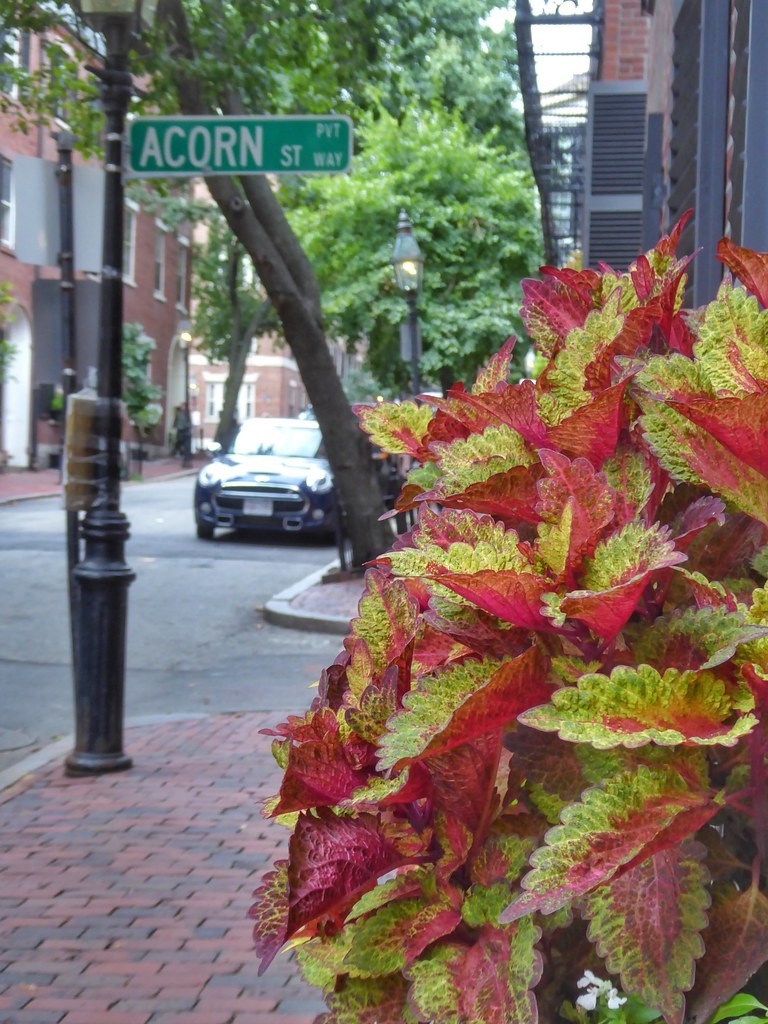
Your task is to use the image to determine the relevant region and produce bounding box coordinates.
[62,0,157,774]
[391,206,429,408]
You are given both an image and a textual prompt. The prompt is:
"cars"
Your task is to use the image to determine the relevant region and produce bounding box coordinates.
[193,402,424,545]
